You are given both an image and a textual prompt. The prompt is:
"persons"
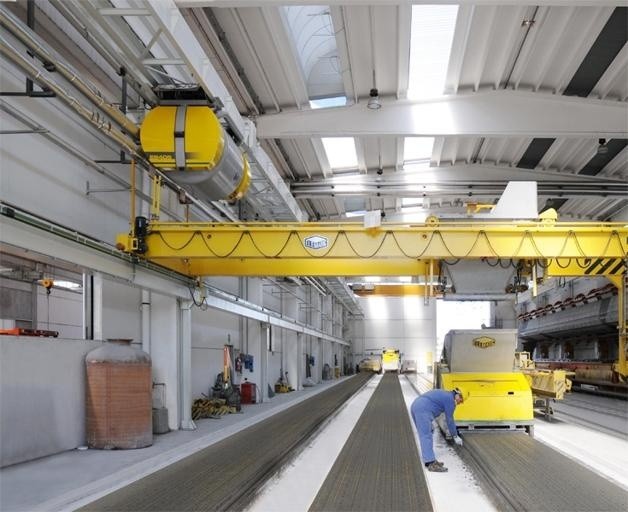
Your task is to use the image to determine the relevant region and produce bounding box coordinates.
[410,384,471,472]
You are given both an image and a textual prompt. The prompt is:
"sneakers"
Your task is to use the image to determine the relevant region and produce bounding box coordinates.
[425,460,448,472]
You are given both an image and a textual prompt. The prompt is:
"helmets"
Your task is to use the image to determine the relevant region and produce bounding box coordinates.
[456,386,470,405]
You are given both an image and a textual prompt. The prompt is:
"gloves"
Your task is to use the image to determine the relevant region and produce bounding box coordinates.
[453,435,463,448]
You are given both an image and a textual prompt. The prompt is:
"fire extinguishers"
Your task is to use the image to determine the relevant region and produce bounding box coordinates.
[236,357,241,371]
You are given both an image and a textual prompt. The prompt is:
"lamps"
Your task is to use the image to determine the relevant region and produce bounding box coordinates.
[597,138,608,155]
[365,70,380,110]
